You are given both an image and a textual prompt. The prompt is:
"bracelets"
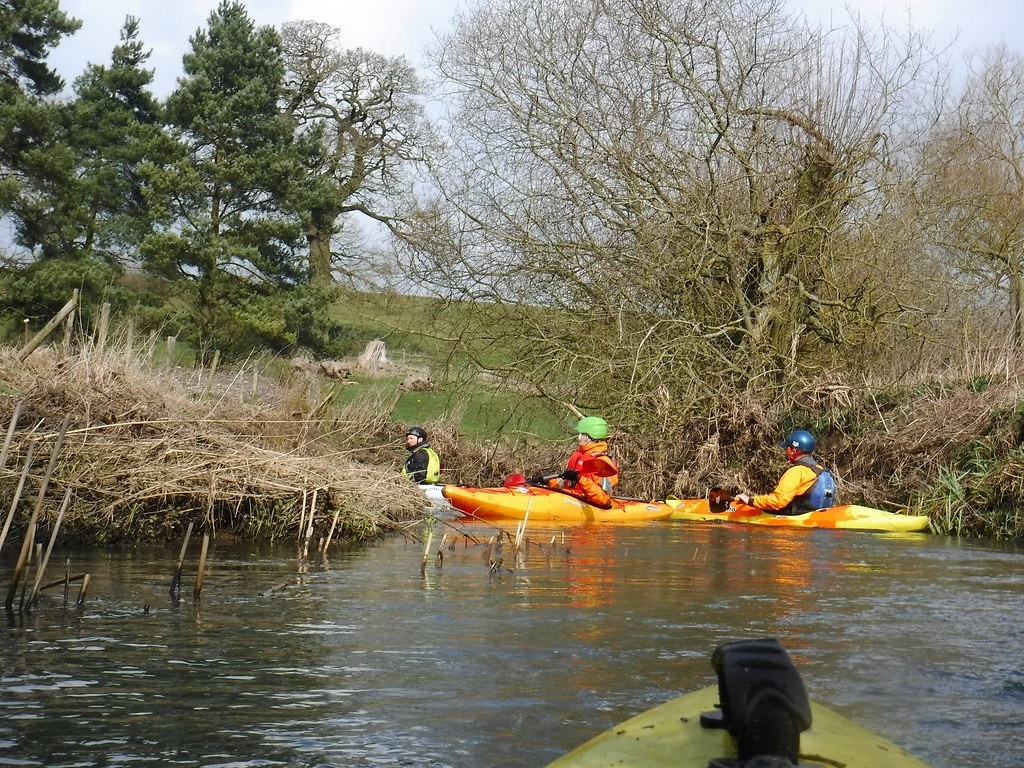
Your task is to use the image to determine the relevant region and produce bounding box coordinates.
[748,495,756,507]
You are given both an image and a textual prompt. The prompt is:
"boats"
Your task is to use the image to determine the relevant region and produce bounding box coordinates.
[454,490,677,524]
[658,494,929,534]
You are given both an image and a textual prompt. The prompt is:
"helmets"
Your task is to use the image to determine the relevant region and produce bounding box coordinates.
[406,427,427,441]
[780,430,815,452]
[574,417,608,441]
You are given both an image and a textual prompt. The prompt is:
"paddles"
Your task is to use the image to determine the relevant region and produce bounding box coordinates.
[503,469,579,488]
[708,485,741,513]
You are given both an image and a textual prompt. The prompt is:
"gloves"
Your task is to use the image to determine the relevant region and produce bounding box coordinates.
[532,476,545,484]
[562,469,580,482]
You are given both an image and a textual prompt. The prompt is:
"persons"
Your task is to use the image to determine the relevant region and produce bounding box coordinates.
[532,415,619,509]
[733,430,836,517]
[401,426,441,484]
